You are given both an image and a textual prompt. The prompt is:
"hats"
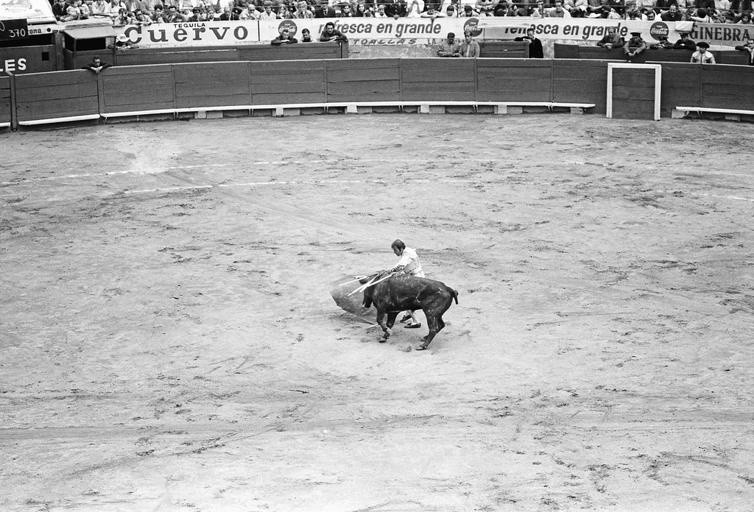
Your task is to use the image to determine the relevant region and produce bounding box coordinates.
[695,41,710,49]
[678,31,690,36]
[658,36,669,41]
[630,31,641,37]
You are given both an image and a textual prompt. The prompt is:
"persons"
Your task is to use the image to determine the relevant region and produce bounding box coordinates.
[434,32,460,57]
[650,34,675,51]
[382,239,426,330]
[689,40,717,65]
[296,28,317,45]
[50,0,754,30]
[736,39,753,67]
[672,31,697,51]
[271,31,298,45]
[623,36,648,59]
[512,27,543,59]
[456,29,480,59]
[318,22,348,45]
[81,56,113,76]
[594,27,624,52]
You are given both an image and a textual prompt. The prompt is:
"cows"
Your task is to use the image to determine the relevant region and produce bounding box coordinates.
[359,271,459,350]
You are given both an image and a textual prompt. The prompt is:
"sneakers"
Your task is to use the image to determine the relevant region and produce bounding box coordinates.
[403,322,422,329]
[399,314,412,323]
